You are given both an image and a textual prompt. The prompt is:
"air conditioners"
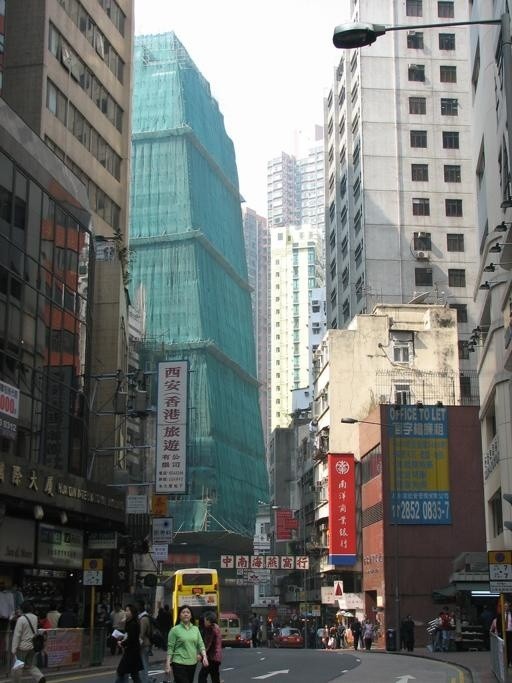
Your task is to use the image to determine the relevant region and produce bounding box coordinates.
[418,232,426,240]
[417,251,429,261]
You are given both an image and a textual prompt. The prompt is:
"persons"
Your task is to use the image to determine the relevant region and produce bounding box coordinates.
[361,616,375,651]
[248,611,262,647]
[350,617,362,650]
[496,599,512,667]
[195,609,224,682]
[45,602,63,628]
[57,603,79,628]
[97,603,172,656]
[479,605,495,650]
[116,603,146,682]
[301,621,353,650]
[488,617,498,636]
[133,601,153,682]
[403,615,415,651]
[11,599,48,683]
[432,606,455,651]
[164,605,209,682]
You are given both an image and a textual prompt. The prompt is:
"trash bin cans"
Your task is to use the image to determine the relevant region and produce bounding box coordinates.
[386,628,396,651]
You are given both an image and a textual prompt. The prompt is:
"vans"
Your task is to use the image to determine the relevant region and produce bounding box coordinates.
[218,613,241,648]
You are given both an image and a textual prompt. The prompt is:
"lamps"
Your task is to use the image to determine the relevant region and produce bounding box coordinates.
[389,401,444,409]
[479,220,512,290]
[467,324,489,353]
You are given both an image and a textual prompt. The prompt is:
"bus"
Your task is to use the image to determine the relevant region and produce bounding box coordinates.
[163,568,220,627]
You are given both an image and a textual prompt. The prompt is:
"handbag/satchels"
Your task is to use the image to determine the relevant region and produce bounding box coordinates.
[32,634,43,651]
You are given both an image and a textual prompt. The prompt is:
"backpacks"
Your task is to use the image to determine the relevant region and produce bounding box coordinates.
[142,614,163,646]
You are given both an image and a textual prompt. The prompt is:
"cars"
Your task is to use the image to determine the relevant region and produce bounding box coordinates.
[232,633,250,648]
[274,627,304,648]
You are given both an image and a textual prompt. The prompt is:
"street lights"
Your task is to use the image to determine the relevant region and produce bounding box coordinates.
[341,417,401,651]
[332,12,512,177]
[272,506,308,649]
